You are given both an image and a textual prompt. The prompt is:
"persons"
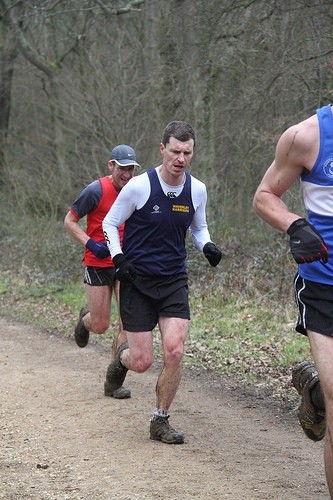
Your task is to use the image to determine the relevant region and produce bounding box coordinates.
[253,105,333,500]
[64,144,141,400]
[102,121,223,445]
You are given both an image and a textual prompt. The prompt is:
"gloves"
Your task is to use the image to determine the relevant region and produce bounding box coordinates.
[203,242,223,267]
[113,254,137,284]
[85,238,110,259]
[286,218,329,264]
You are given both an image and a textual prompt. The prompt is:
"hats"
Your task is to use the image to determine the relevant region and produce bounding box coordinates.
[109,144,141,168]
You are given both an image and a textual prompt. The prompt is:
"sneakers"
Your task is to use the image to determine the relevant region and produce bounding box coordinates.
[148,414,184,443]
[75,308,91,348]
[104,382,130,398]
[105,343,129,390]
[293,361,326,441]
